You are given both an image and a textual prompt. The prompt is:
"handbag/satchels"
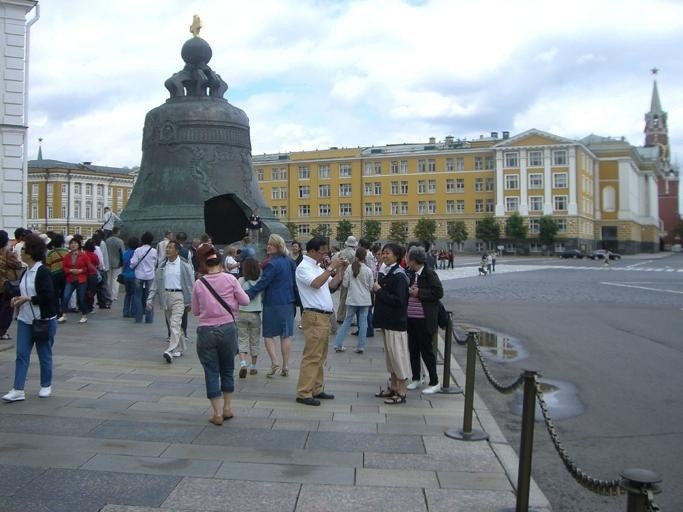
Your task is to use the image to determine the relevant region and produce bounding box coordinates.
[33,319,50,338]
[117,275,124,283]
[6,280,19,296]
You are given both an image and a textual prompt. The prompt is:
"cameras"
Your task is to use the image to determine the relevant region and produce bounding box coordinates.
[339,256,348,266]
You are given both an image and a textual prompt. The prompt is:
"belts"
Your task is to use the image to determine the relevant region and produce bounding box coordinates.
[306,308,333,314]
[165,288,182,292]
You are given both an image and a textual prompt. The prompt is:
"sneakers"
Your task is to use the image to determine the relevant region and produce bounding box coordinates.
[57,316,67,321]
[164,351,182,362]
[80,317,88,323]
[1,389,25,401]
[239,366,288,378]
[375,381,440,403]
[209,409,233,424]
[38,386,52,397]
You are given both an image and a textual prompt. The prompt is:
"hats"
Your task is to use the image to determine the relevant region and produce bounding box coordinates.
[345,236,358,246]
[64,236,73,247]
[39,233,52,245]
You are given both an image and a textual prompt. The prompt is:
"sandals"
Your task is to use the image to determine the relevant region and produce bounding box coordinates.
[0,334,12,340]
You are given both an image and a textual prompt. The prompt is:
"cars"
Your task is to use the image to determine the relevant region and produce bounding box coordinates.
[559,250,621,260]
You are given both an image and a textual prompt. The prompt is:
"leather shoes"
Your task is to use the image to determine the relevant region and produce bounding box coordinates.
[296,392,334,405]
[336,330,363,353]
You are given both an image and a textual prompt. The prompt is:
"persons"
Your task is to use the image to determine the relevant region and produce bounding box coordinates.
[2,228,382,352]
[146,242,192,364]
[1,234,56,400]
[372,244,444,403]
[191,245,250,424]
[98,207,120,240]
[245,234,296,378]
[433,248,455,269]
[603,250,609,266]
[295,238,348,406]
[237,258,262,378]
[478,251,497,275]
